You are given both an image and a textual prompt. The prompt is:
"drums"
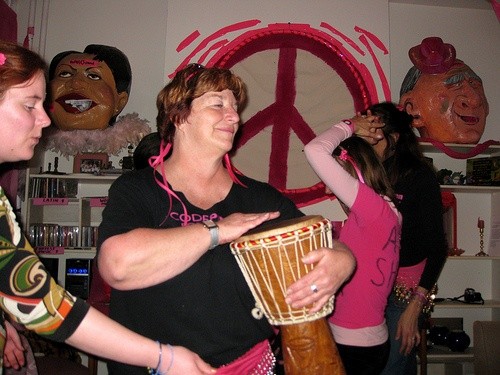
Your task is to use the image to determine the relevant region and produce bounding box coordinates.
[229,215,345,375]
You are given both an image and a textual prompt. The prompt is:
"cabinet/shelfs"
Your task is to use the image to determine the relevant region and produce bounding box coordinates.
[23,143,136,375]
[404,142,500,375]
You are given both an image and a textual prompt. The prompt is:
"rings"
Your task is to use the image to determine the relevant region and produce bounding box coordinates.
[310,285,317,294]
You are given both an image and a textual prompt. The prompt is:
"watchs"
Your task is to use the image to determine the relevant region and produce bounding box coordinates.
[201,220,219,250]
[341,118,355,136]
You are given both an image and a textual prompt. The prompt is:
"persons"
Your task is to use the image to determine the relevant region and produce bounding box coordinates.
[303,109,403,375]
[92,62,357,375]
[397,36,491,145]
[0,45,216,375]
[358,101,448,375]
[47,42,133,132]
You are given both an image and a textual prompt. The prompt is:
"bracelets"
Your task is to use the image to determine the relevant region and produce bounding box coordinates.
[147,340,163,375]
[407,289,438,315]
[165,344,175,375]
